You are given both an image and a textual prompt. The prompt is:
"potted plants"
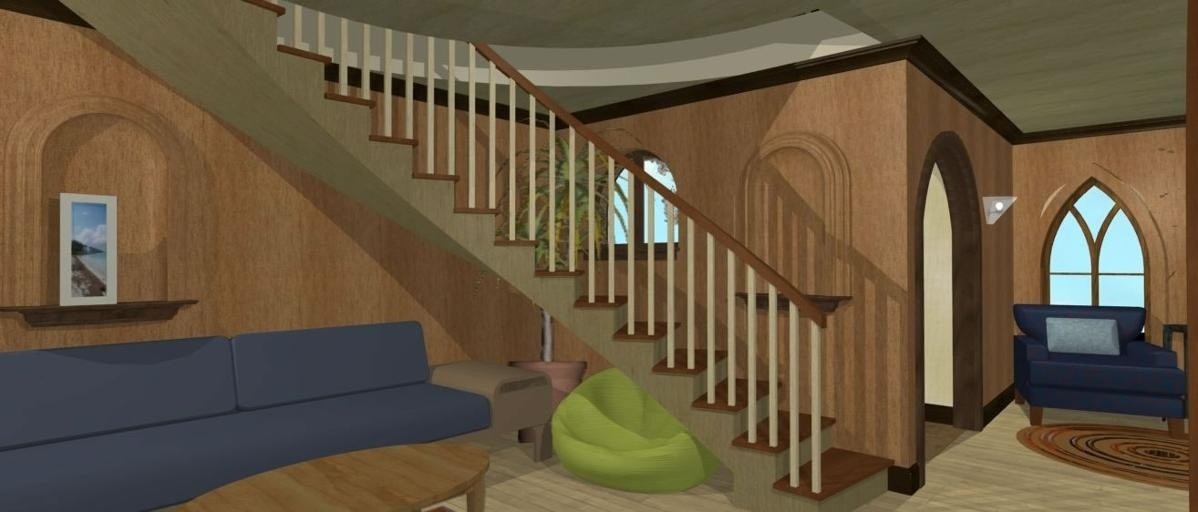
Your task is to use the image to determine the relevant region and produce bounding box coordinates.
[495,116,644,443]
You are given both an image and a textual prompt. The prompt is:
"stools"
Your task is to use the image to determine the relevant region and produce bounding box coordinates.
[550,367,716,494]
[431,360,552,461]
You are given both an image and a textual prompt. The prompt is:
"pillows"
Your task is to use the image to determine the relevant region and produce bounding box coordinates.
[1046,317,1120,356]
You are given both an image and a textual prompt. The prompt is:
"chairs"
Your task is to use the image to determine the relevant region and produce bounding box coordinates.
[1012,303,1186,438]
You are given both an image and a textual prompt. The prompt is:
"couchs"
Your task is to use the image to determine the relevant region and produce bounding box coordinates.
[0,319,494,512]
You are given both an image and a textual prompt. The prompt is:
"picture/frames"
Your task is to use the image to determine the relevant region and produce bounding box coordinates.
[60,193,118,306]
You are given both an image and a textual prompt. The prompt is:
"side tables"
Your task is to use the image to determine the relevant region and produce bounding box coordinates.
[172,443,490,512]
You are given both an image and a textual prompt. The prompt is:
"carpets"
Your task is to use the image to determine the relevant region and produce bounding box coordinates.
[1018,422,1189,491]
